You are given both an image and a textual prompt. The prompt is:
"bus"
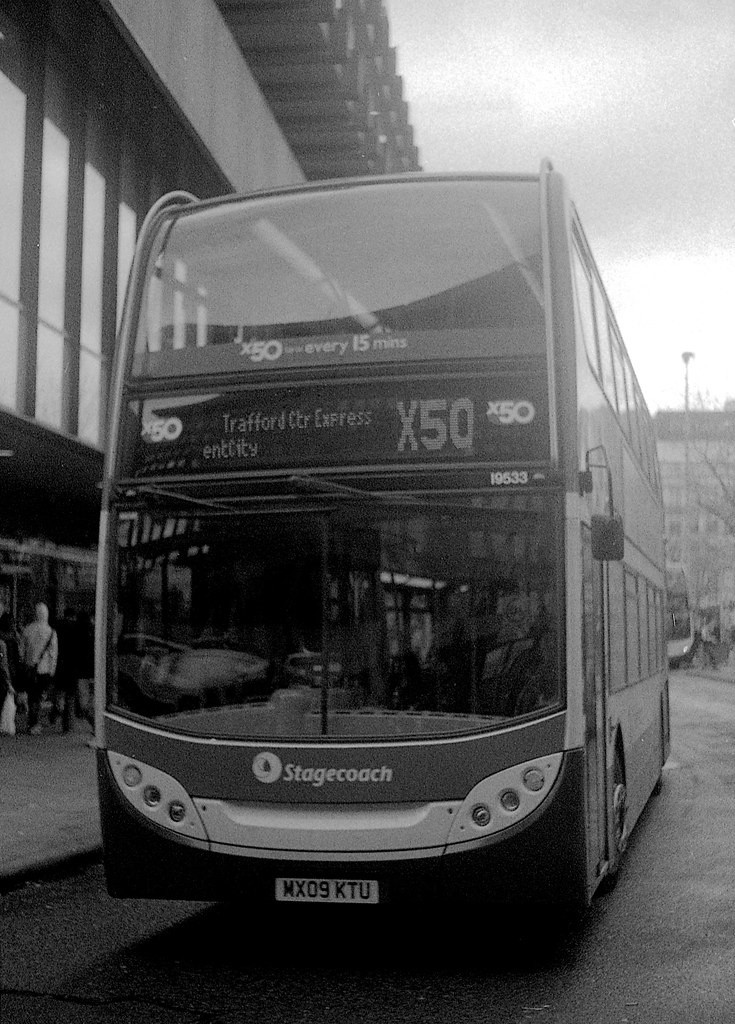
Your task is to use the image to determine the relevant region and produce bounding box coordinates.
[666,563,694,657]
[95,157,671,909]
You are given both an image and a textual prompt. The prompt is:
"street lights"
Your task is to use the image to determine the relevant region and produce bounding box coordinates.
[683,352,694,475]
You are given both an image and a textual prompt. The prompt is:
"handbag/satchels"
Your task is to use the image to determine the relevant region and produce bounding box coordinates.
[0,691,17,736]
[17,664,39,684]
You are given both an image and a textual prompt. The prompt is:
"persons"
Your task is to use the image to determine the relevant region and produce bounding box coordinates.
[682,596,724,671]
[403,556,556,716]
[0,600,95,736]
